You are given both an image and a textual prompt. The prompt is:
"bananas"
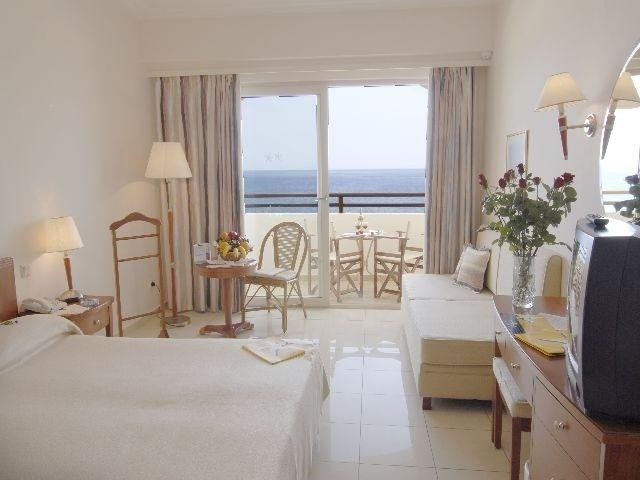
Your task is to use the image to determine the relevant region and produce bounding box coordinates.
[222,245,246,258]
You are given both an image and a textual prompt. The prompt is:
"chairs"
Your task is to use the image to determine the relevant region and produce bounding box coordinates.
[303,233,364,301]
[244,220,308,333]
[372,230,427,303]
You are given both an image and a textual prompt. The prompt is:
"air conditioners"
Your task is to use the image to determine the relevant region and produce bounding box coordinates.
[601,72,640,158]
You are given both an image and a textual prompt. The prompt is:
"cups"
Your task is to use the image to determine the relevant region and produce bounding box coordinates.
[192,244,207,264]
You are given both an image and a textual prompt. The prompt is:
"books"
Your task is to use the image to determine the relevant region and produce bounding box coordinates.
[515,314,559,332]
[514,329,565,357]
[241,335,306,365]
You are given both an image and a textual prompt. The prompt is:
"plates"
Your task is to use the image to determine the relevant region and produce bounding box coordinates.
[207,260,224,265]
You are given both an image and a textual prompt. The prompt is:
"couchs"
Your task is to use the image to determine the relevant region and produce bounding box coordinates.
[399,221,563,410]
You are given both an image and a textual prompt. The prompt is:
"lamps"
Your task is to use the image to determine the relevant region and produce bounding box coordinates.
[144,142,194,328]
[43,214,86,300]
[535,70,597,159]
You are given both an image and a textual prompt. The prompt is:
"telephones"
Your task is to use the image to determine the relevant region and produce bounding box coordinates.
[22,297,67,314]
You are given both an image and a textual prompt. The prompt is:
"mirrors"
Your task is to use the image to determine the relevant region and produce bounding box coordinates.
[597,40,640,228]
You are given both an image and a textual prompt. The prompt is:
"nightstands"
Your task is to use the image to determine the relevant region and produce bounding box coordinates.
[20,294,116,337]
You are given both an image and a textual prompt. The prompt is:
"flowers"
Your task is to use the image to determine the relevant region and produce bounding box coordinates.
[614,170,640,224]
[473,164,577,298]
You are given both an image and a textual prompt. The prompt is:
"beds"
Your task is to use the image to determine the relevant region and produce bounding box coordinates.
[2,257,330,480]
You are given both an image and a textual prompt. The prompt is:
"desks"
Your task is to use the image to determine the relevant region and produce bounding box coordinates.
[341,231,386,282]
[194,259,255,339]
[489,293,639,480]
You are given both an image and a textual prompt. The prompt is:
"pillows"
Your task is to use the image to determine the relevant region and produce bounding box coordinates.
[453,246,492,293]
[450,242,470,282]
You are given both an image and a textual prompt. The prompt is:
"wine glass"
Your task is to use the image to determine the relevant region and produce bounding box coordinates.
[355,222,368,235]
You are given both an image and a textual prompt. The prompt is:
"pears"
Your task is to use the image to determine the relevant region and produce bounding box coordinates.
[220,232,228,241]
[240,242,249,251]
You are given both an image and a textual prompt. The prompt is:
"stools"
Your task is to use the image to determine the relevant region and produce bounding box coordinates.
[489,356,532,479]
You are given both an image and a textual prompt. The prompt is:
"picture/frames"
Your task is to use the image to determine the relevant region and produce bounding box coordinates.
[504,129,527,171]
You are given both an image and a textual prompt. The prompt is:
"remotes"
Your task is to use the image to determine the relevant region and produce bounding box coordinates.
[586,213,609,225]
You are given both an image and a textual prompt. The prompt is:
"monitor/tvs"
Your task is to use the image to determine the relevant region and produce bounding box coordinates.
[564,213,640,420]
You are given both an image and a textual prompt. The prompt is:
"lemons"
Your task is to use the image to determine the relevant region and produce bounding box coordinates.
[219,242,229,250]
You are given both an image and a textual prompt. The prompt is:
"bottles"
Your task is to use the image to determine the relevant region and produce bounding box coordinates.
[358,207,364,222]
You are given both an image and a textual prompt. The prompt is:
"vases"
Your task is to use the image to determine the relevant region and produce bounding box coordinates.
[509,253,537,308]
